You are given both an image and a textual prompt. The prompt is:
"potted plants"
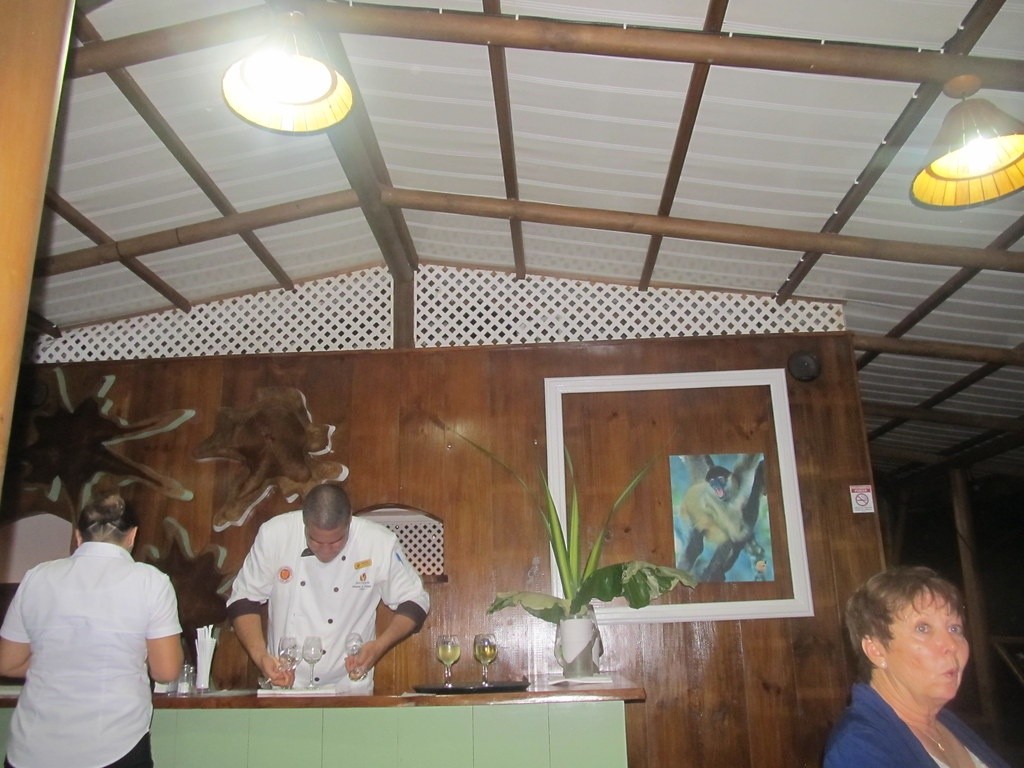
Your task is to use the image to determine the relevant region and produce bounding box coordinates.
[454,419,696,677]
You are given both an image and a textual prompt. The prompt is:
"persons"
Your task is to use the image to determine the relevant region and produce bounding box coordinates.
[0,493,186,768]
[818,565,1015,768]
[225,482,429,697]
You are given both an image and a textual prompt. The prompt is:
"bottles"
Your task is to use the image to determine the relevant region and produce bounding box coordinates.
[191,667,196,696]
[176,664,192,698]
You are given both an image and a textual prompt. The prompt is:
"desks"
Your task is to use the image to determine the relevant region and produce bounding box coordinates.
[1,675,647,768]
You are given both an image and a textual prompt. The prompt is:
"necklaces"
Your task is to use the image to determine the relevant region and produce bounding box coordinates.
[894,708,945,752]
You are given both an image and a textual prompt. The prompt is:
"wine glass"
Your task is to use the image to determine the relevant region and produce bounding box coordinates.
[277,637,298,690]
[302,636,323,690]
[257,647,303,690]
[435,635,461,689]
[346,633,367,682]
[473,633,497,688]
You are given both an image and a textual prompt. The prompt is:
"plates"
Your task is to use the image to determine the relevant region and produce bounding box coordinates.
[413,681,531,694]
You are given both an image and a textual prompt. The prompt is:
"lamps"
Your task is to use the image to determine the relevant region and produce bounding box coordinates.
[219,6,354,138]
[909,71,1024,210]
